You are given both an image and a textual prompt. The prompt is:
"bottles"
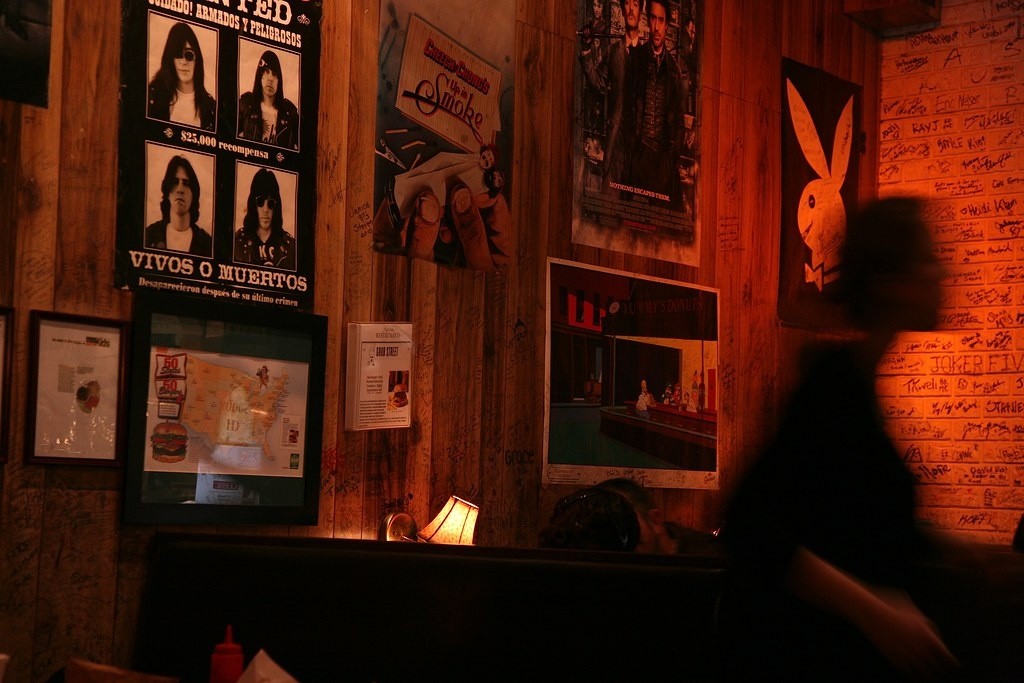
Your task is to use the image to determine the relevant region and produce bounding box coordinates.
[210,624,242,682]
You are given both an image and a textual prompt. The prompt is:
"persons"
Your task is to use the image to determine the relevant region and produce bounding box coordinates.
[719,197,962,683]
[580,0,698,214]
[148,21,218,132]
[537,490,642,553]
[238,51,299,150]
[235,168,295,269]
[144,156,212,256]
[598,478,718,553]
[639,380,676,405]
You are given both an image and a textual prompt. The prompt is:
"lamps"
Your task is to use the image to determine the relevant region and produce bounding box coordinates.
[415,494,480,545]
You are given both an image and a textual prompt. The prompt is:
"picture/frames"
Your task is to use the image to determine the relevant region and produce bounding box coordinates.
[22,309,126,471]
[118,289,329,529]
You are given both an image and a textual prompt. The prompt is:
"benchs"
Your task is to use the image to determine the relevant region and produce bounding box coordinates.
[129,529,738,683]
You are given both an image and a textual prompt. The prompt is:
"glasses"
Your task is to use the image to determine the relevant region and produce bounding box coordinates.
[256,196,277,209]
[174,49,195,61]
